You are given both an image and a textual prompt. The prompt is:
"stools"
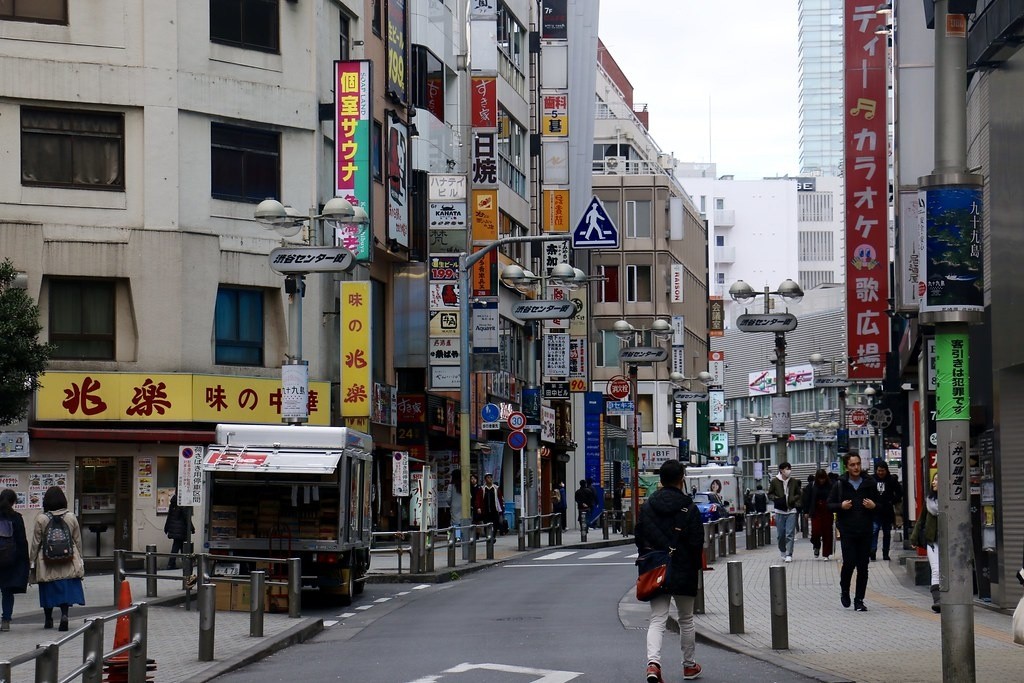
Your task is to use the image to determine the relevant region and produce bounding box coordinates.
[89,525,107,557]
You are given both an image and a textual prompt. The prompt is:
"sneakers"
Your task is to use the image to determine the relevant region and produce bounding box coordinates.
[840,590,851,607]
[646,666,664,683]
[683,664,702,679]
[854,599,867,612]
[780,551,786,560]
[786,556,792,562]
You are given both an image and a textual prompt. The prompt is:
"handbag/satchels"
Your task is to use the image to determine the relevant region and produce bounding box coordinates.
[634,550,679,602]
[499,514,509,536]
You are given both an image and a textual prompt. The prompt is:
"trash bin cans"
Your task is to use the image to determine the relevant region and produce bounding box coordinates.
[504,502,515,530]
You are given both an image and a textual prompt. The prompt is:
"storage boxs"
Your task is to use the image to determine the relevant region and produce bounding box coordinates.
[210,560,288,612]
[210,500,338,541]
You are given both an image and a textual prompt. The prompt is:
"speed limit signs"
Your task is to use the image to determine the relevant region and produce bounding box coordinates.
[506,411,527,431]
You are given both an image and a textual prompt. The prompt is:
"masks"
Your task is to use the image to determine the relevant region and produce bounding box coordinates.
[781,468,792,477]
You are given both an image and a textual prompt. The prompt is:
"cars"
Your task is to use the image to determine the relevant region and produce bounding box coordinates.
[687,491,729,524]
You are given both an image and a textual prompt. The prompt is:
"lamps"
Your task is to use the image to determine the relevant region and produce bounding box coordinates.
[408,185,417,197]
[408,104,417,117]
[388,238,400,252]
[447,159,455,168]
[475,131,479,139]
[388,174,400,183]
[388,90,400,105]
[406,247,420,263]
[459,140,463,147]
[408,123,419,138]
[388,109,401,124]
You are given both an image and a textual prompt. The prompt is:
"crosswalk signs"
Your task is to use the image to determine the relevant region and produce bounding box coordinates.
[571,193,621,250]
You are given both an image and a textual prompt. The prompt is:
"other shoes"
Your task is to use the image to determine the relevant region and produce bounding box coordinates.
[0,621,10,631]
[814,548,892,561]
[562,527,569,533]
[59,616,68,631]
[456,539,462,547]
[493,537,496,544]
[167,564,179,570]
[44,618,53,628]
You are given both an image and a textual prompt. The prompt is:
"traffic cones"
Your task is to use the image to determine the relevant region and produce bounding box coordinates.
[102,579,157,683]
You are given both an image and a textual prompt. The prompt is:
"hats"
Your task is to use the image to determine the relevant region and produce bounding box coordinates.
[484,473,493,479]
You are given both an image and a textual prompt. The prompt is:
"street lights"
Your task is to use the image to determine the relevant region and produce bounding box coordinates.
[668,371,715,468]
[728,277,805,465]
[500,262,589,549]
[612,319,676,526]
[253,195,372,426]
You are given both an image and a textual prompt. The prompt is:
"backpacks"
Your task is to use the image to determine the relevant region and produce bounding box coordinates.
[40,511,75,563]
[0,512,18,559]
[550,487,561,503]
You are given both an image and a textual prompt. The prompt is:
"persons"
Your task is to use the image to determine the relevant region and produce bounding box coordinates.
[575,479,625,533]
[164,488,195,569]
[0,489,31,631]
[711,480,723,499]
[446,469,506,542]
[910,473,940,613]
[30,487,85,631]
[870,461,900,561]
[553,480,570,532]
[635,460,705,683]
[826,453,880,611]
[745,462,839,562]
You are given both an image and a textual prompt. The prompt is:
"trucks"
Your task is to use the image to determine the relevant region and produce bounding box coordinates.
[683,465,745,532]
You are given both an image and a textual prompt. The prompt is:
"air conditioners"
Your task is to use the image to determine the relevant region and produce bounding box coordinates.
[605,156,625,175]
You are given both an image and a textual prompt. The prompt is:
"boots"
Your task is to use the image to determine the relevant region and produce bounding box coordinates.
[930,584,941,612]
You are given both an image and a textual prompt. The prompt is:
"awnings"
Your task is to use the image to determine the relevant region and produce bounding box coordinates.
[204,448,341,474]
